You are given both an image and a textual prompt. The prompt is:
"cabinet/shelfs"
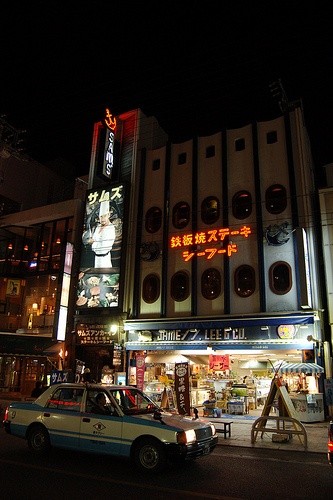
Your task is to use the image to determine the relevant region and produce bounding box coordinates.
[0,277,26,332]
[131,359,278,418]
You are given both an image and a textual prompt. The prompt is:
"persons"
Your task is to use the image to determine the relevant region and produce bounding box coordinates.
[31,381,44,398]
[94,392,112,416]
[90,199,114,267]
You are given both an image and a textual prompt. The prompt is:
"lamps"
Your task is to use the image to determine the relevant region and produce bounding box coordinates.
[3,234,62,262]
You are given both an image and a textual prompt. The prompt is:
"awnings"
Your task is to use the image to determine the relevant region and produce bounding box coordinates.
[270,361,325,374]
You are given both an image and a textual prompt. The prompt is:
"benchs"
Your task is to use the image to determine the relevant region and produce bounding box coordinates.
[208,421,232,439]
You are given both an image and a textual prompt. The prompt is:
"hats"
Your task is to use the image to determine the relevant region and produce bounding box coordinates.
[98,201,109,216]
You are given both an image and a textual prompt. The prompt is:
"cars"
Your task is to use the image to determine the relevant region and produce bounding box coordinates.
[3,382,219,475]
